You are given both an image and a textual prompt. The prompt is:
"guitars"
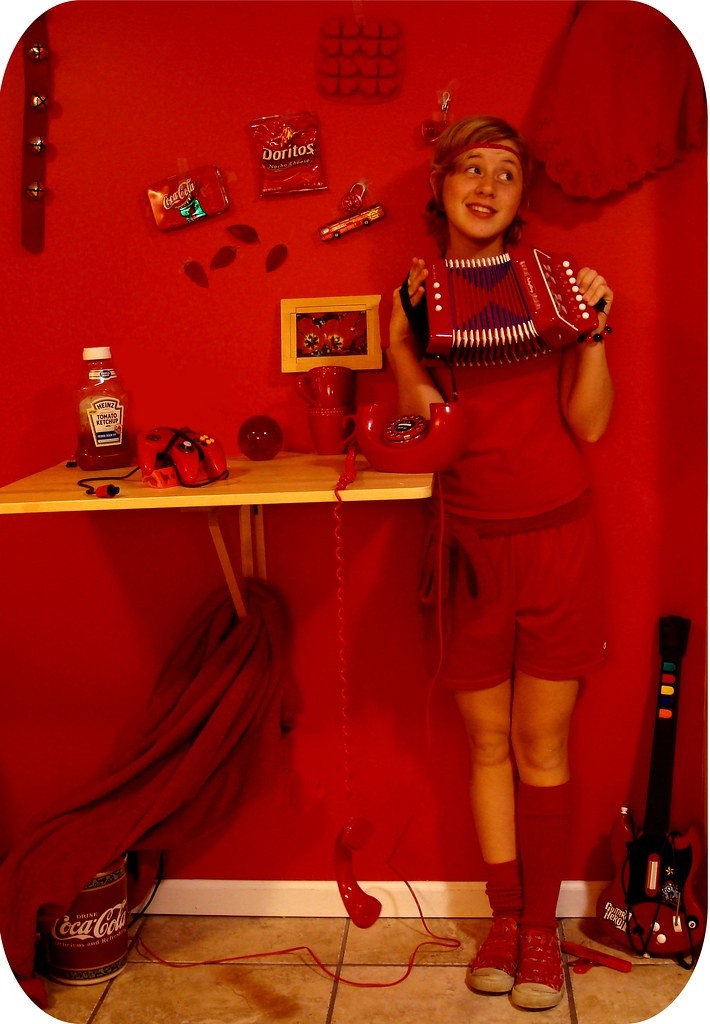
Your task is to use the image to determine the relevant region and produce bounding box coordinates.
[595,615,706,959]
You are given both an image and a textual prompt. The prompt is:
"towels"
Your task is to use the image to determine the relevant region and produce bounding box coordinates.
[0,579,304,1009]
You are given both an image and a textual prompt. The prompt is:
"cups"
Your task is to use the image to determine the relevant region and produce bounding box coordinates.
[307,409,358,455]
[296,366,353,408]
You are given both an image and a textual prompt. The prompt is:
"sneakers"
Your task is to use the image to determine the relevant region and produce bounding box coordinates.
[466,912,520,994]
[509,924,566,1010]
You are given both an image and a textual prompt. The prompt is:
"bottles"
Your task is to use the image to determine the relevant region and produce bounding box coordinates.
[73,347,131,471]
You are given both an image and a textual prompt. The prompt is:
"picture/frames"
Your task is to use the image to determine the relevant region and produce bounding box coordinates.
[281,295,383,373]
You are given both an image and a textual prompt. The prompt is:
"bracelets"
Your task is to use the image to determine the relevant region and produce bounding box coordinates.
[577,324,613,344]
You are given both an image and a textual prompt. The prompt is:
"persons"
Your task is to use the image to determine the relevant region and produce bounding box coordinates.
[387,117,613,1008]
